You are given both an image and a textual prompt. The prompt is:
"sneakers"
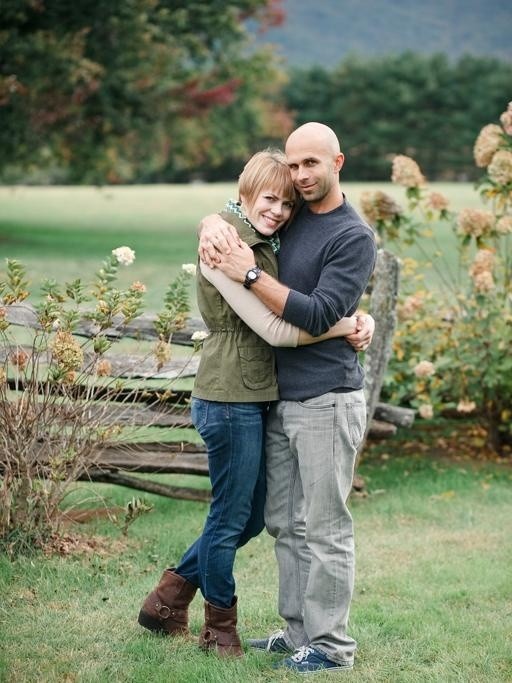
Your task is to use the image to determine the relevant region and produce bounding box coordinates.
[245,632,290,654]
[272,645,353,674]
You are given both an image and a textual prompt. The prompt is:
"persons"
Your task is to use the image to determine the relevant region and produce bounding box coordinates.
[194,120,377,676]
[136,146,377,663]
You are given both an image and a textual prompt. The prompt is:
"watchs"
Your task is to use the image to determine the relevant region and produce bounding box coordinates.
[242,264,263,290]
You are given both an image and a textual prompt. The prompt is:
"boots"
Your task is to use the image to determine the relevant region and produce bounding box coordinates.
[199,596,243,660]
[138,568,198,642]
